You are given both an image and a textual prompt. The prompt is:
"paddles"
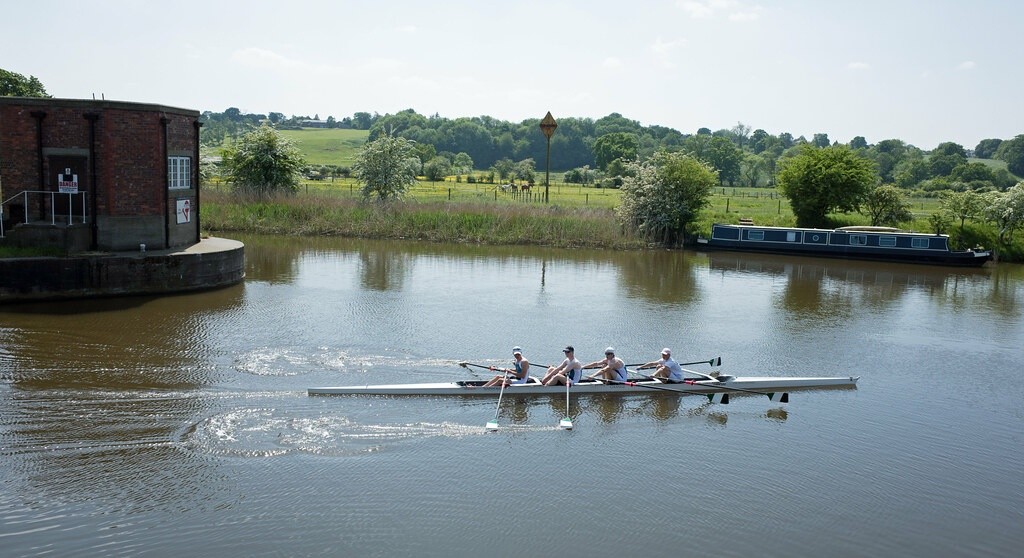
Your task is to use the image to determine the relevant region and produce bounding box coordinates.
[511,359,551,369]
[484,367,509,432]
[584,375,731,405]
[636,355,723,372]
[558,370,574,430]
[650,376,791,405]
[455,360,508,373]
[580,362,647,371]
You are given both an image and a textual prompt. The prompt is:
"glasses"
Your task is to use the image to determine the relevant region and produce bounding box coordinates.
[606,354,613,356]
[514,354,520,356]
[564,351,572,353]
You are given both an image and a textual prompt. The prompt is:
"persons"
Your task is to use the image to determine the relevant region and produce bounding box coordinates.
[482,346,530,387]
[540,347,583,392]
[584,347,628,384]
[637,348,683,384]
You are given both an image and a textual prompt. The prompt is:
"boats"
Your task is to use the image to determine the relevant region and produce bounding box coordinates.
[306,374,861,396]
[695,224,992,267]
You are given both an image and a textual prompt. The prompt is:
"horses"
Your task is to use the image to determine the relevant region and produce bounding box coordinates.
[502,183,533,192]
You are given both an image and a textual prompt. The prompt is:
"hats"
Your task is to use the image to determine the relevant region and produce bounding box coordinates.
[563,346,574,352]
[605,347,615,353]
[661,347,671,355]
[512,346,522,354]
